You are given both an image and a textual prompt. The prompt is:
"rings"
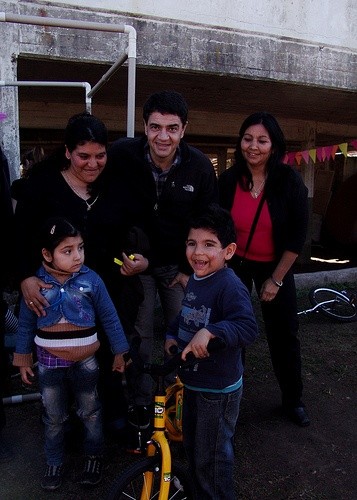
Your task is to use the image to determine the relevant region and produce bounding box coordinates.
[30,301,33,305]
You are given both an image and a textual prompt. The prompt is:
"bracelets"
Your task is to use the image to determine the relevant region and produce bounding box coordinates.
[270,274,283,287]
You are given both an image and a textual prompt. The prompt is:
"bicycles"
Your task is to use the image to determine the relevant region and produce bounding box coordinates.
[103,351,202,500]
[297,284,357,324]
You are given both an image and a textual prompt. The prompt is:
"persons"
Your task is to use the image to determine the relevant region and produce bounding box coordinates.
[0,147,13,431]
[214,110,312,427]
[13,218,130,490]
[164,206,258,500]
[90,90,218,369]
[14,112,108,318]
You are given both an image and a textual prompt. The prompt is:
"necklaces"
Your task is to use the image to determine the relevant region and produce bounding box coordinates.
[67,172,101,211]
[250,178,265,198]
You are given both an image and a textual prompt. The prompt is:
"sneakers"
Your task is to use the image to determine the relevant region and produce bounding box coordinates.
[40,462,64,490]
[80,457,104,485]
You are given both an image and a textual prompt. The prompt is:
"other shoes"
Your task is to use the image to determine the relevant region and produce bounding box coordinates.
[288,406,310,427]
[0,434,14,463]
[128,405,150,429]
